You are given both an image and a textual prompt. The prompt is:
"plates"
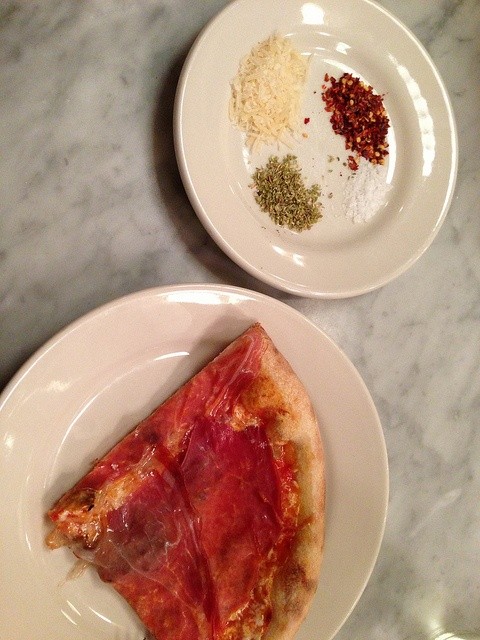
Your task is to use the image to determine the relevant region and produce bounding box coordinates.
[0,283,392,640]
[171,0,458,301]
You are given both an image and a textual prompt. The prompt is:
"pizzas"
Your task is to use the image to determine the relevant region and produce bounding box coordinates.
[44,321,327,639]
